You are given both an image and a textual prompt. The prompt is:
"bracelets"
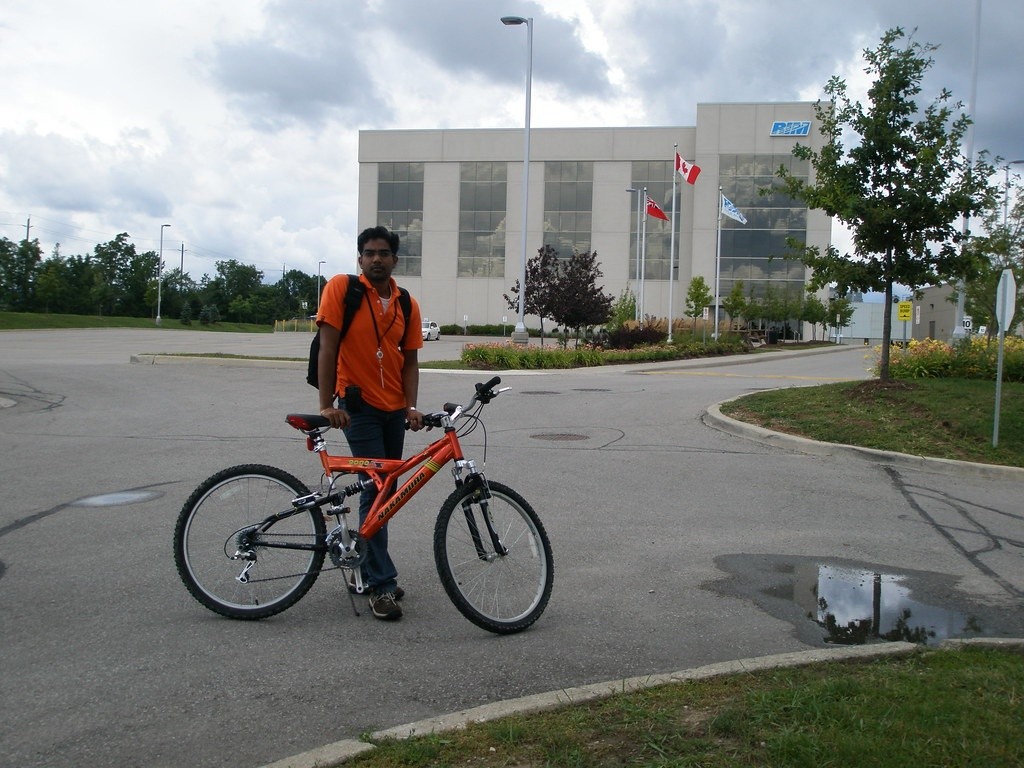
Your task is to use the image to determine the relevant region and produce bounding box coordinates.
[406,407,416,412]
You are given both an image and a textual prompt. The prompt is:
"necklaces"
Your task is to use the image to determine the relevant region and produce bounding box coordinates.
[360,280,397,389]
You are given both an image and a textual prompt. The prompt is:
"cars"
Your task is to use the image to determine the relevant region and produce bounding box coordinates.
[421,321,442,341]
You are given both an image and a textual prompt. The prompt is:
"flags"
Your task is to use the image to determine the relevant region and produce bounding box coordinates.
[675,151,701,185]
[721,193,747,224]
[646,194,669,221]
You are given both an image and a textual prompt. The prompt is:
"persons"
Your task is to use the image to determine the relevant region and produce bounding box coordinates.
[315,225,425,620]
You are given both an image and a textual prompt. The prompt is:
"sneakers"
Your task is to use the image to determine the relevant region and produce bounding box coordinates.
[348,570,402,601]
[367,591,403,620]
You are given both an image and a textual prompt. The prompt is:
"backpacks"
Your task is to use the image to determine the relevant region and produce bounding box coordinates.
[306,274,411,394]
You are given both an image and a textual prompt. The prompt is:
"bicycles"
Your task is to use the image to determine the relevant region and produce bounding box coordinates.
[172,374,555,634]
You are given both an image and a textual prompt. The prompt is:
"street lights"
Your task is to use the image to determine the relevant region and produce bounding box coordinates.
[1003,160,1023,236]
[501,13,534,348]
[318,261,326,311]
[624,188,642,325]
[156,224,172,319]
